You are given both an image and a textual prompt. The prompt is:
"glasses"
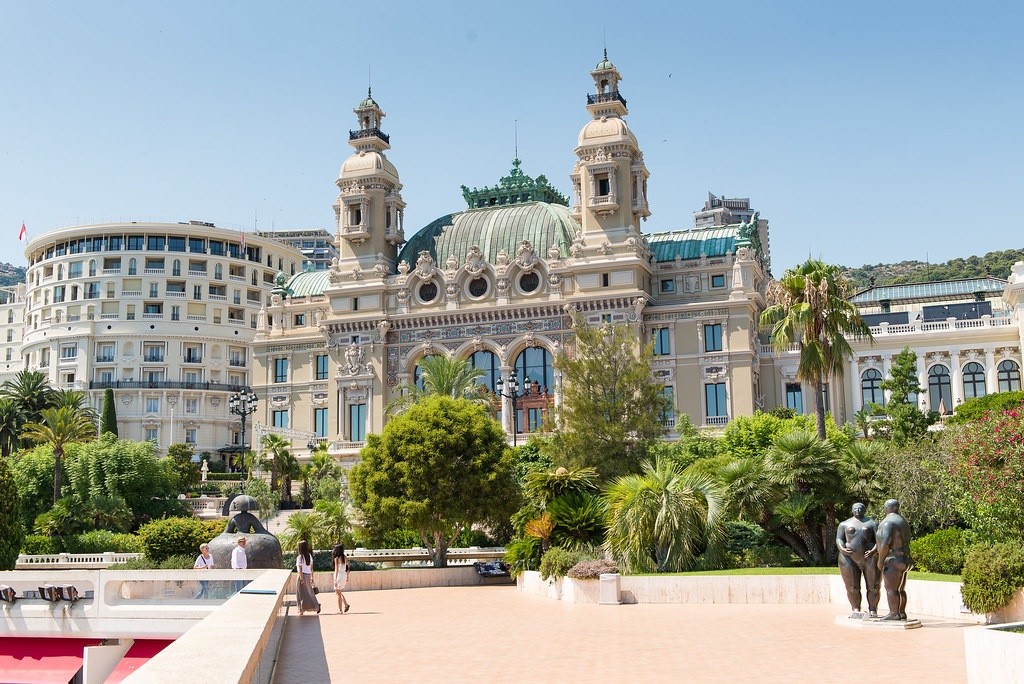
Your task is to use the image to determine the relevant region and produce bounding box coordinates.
[202,547,210,549]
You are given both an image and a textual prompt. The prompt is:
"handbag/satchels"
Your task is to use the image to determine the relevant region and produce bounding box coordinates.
[311,581,319,595]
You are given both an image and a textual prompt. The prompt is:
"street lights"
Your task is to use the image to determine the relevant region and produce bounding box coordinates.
[229,389,259,490]
[497,371,532,446]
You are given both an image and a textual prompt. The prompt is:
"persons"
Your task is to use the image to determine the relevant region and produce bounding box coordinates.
[192,542,215,599]
[874,498,914,621]
[223,537,248,601]
[836,501,882,620]
[331,543,350,614]
[295,539,322,616]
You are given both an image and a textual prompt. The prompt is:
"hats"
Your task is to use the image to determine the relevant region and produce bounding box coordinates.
[237,536,247,542]
